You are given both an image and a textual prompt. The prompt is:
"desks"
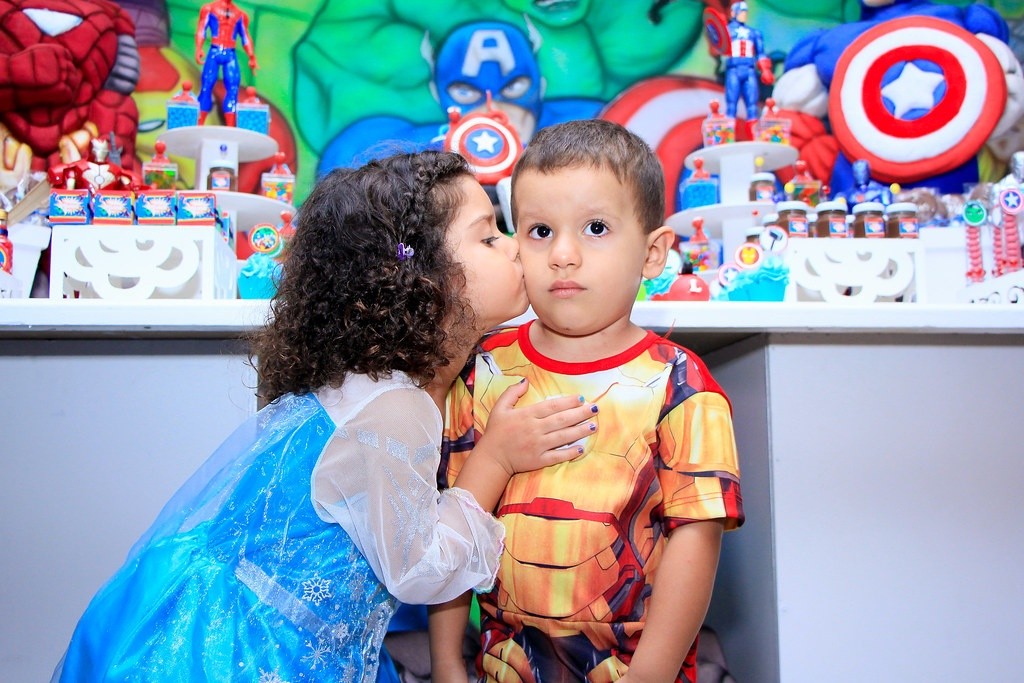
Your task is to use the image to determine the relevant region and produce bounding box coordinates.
[2,298,1024,681]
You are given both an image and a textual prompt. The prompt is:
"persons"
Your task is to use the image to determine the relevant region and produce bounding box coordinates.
[50,147,599,683]
[841,160,892,215]
[424,121,746,683]
[196,0,260,126]
[717,1,775,122]
